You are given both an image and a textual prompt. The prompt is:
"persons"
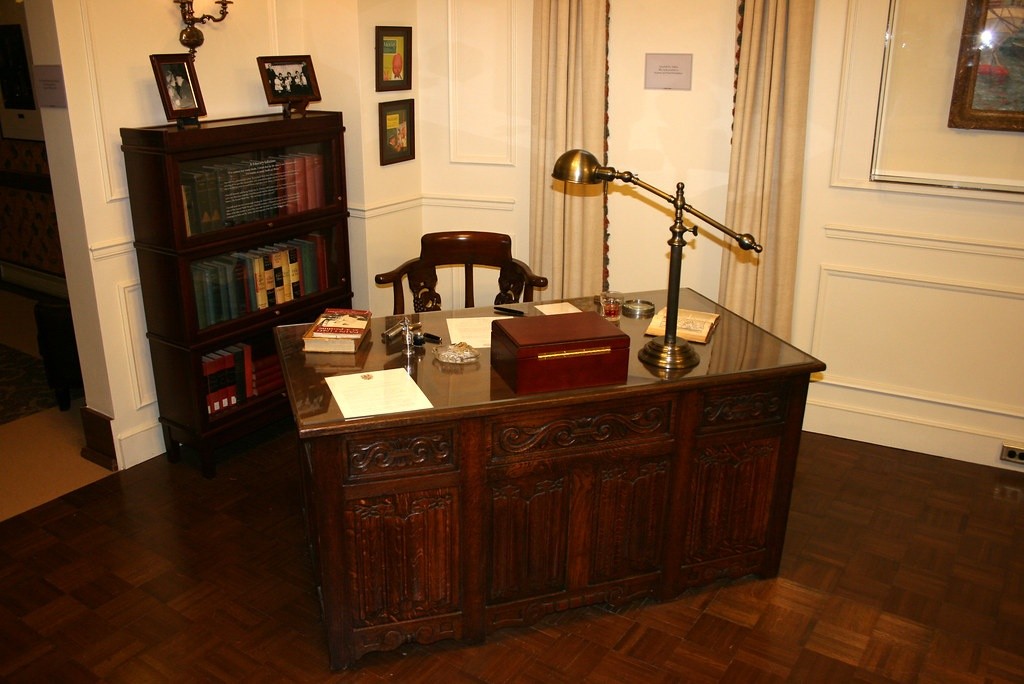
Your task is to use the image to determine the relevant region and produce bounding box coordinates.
[166,72,195,110]
[387,134,404,156]
[265,62,314,97]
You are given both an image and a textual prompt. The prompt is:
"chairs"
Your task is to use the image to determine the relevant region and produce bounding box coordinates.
[375,230,548,316]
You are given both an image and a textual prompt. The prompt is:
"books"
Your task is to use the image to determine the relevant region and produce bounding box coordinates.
[201,343,284,414]
[191,232,329,329]
[179,151,326,239]
[644,305,720,344]
[301,308,373,353]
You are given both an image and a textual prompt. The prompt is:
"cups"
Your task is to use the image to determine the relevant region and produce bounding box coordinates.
[598,289,624,322]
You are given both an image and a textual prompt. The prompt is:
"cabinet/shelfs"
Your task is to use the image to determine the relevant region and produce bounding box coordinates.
[120,111,354,480]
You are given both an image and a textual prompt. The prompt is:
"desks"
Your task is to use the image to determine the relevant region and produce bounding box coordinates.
[273,289,826,673]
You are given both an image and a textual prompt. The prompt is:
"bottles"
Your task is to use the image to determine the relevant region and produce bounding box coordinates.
[401,316,416,356]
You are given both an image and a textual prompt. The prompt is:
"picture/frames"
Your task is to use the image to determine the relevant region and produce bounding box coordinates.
[379,99,415,166]
[375,27,412,92]
[948,0,1024,132]
[257,55,322,106]
[149,53,207,121]
